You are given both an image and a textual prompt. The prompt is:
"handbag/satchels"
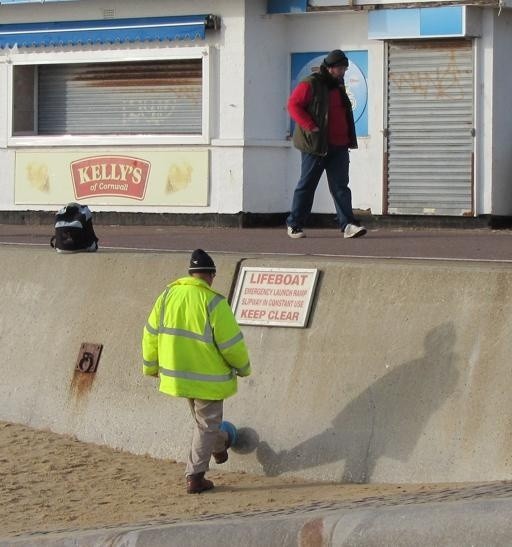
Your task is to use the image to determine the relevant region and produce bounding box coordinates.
[51,202,99,253]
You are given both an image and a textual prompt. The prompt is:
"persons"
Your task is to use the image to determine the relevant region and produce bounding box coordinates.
[284,49,368,240]
[141,248,251,494]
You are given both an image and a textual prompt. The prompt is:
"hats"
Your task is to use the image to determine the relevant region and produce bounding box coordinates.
[323,50,348,67]
[189,249,216,273]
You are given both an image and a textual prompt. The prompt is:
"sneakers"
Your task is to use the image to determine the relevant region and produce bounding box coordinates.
[213,432,232,464]
[287,224,304,238]
[186,473,213,494]
[344,224,366,238]
